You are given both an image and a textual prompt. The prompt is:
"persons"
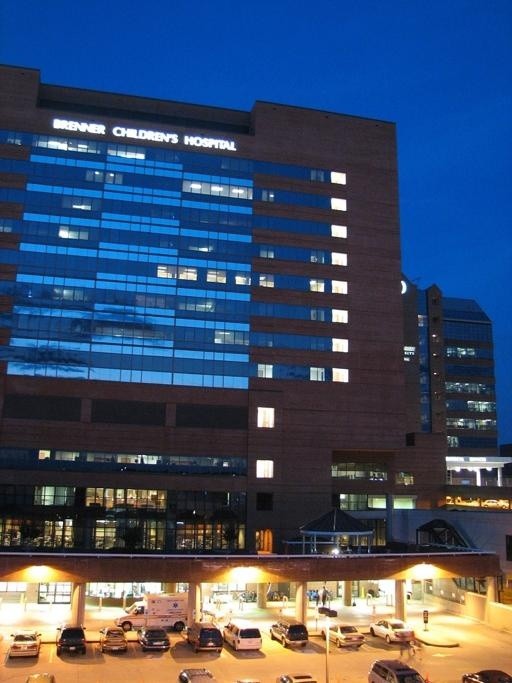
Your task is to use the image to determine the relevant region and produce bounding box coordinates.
[314,590,320,607]
[322,586,328,606]
[242,590,256,602]
[268,590,285,601]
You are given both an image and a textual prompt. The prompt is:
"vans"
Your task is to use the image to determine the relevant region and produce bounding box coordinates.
[223,618,262,651]
[368,658,425,682]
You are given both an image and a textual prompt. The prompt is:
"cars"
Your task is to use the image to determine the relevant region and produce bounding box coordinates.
[9,630,42,659]
[321,623,365,648]
[137,625,170,652]
[462,669,511,682]
[26,672,55,683]
[179,667,217,683]
[56,623,87,656]
[279,672,317,682]
[369,618,415,644]
[100,625,127,653]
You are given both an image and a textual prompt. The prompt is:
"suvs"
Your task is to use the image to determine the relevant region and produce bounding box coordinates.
[187,622,223,652]
[269,618,308,647]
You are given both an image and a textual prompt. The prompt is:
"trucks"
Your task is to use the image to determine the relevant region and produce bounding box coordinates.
[115,592,187,631]
